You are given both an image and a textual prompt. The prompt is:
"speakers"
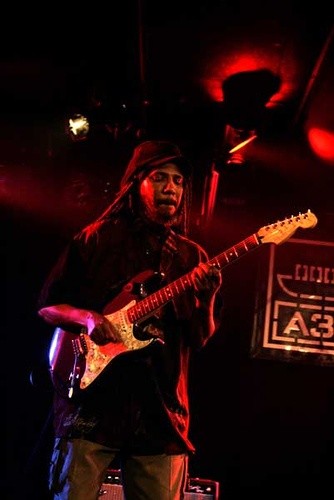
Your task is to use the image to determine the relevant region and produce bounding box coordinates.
[98,468,220,500]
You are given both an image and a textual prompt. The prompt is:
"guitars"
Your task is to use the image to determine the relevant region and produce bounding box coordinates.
[49,210,318,393]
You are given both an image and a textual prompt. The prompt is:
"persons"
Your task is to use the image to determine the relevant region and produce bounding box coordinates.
[37,139,224,500]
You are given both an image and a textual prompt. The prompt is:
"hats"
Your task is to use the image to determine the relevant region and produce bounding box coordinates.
[123,140,193,181]
